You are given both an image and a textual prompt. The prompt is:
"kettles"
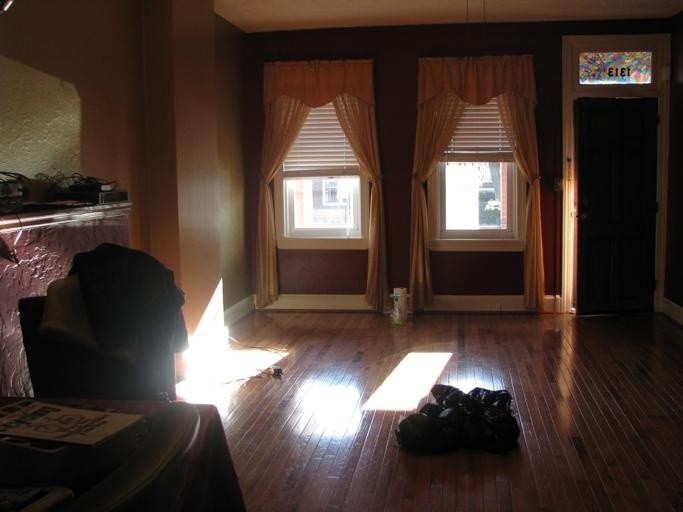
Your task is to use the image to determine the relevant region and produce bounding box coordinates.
[393,286,412,321]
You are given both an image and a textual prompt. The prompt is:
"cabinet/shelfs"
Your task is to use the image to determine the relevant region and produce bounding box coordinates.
[2,201,132,398]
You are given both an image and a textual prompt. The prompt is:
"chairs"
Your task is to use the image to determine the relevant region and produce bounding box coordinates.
[18,296,177,402]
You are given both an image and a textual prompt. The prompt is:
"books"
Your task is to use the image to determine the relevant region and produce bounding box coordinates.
[2,398,143,447]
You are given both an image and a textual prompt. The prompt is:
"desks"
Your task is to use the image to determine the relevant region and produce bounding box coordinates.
[0,396,217,512]
